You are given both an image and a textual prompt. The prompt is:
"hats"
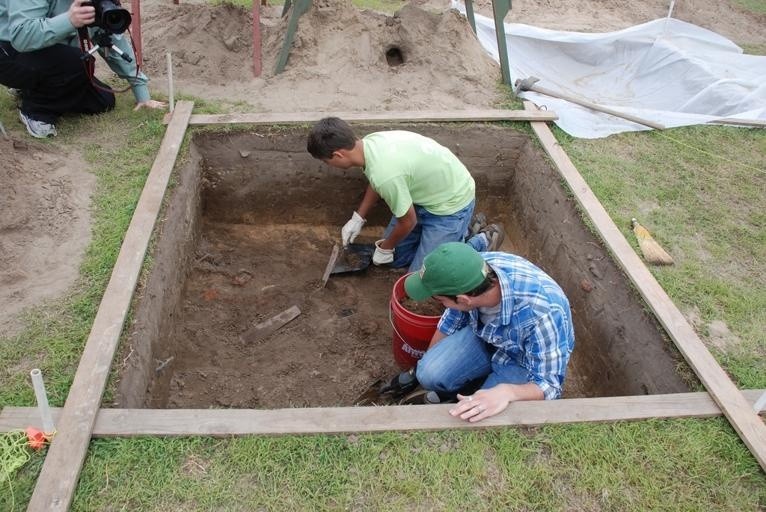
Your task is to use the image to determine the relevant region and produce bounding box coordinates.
[404,242,489,301]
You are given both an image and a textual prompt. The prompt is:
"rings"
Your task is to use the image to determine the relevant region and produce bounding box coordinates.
[468,395,472,401]
[477,406,482,413]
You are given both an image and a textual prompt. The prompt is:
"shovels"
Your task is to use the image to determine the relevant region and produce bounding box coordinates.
[330,242,375,274]
[352,378,405,407]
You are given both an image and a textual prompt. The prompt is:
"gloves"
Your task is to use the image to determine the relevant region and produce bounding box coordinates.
[372,239,396,266]
[342,211,367,247]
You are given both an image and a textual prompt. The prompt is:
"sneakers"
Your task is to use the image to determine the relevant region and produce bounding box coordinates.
[423,385,470,404]
[18,109,58,139]
[465,212,504,251]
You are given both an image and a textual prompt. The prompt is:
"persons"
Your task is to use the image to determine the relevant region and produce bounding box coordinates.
[307,116,505,275]
[0,0,169,140]
[378,242,574,423]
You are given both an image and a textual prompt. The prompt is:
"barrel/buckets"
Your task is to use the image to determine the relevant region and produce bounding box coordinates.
[389,271,443,371]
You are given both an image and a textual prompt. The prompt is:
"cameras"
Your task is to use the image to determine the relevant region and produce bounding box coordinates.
[80,0,131,34]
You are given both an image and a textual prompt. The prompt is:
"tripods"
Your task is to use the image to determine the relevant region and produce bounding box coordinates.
[80,39,132,62]
